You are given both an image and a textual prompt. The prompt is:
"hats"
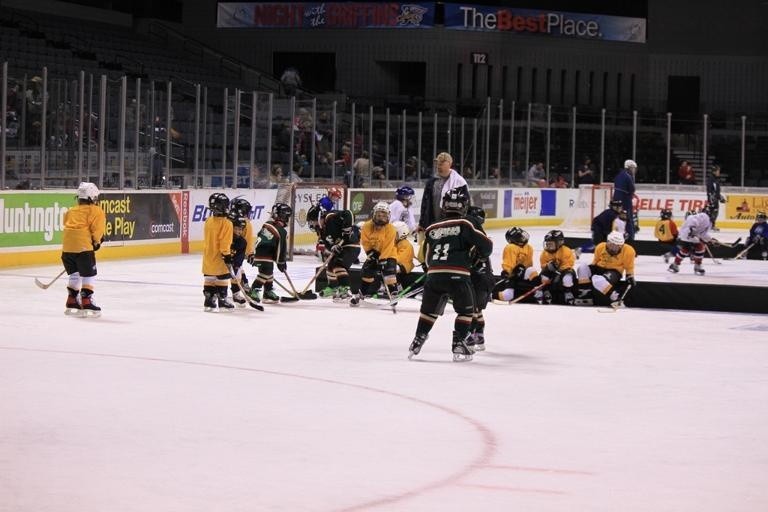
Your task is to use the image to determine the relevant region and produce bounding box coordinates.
[433,152,452,164]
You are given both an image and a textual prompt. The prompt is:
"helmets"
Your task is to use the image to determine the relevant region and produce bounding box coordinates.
[328,187,341,199]
[468,206,484,223]
[394,185,413,202]
[230,199,250,214]
[208,193,229,215]
[371,201,391,226]
[271,203,292,222]
[227,208,246,228]
[623,160,637,168]
[442,188,468,216]
[704,204,717,220]
[660,209,671,218]
[392,221,408,241]
[77,182,98,204]
[685,210,696,218]
[606,230,624,245]
[609,199,622,206]
[306,205,327,232]
[756,211,766,220]
[505,227,529,246]
[542,230,564,251]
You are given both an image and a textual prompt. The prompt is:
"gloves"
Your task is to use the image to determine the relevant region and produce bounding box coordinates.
[547,260,559,271]
[377,259,387,270]
[501,271,509,283]
[277,259,286,272]
[625,274,636,287]
[223,255,231,264]
[341,227,353,240]
[92,241,99,251]
[246,254,255,267]
[550,271,561,284]
[366,249,378,262]
[330,245,343,255]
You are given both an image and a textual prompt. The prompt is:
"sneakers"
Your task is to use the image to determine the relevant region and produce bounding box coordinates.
[576,288,593,299]
[543,291,551,300]
[609,290,623,305]
[318,283,337,297]
[231,287,245,304]
[203,290,216,308]
[65,287,80,309]
[263,285,279,300]
[350,294,359,305]
[80,288,100,310]
[215,291,234,308]
[669,263,678,272]
[693,264,704,272]
[564,291,573,303]
[408,330,427,355]
[240,279,250,292]
[468,328,484,344]
[247,282,261,302]
[332,285,352,298]
[451,330,474,355]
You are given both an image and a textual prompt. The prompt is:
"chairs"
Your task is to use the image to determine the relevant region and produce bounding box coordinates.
[715,142,768,185]
[0,7,138,80]
[7,81,215,167]
[141,24,228,80]
[215,79,355,177]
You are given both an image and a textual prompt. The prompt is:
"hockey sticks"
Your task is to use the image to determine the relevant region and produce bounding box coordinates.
[381,271,396,314]
[234,275,264,312]
[271,241,344,302]
[728,240,755,260]
[494,278,552,305]
[597,285,631,312]
[706,244,722,264]
[390,273,426,305]
[35,269,66,289]
[715,237,742,248]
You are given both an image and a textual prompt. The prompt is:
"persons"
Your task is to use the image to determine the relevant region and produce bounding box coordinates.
[0,66,190,191]
[408,187,493,356]
[60,181,107,311]
[201,151,642,310]
[463,205,496,347]
[190,61,768,189]
[654,160,768,275]
